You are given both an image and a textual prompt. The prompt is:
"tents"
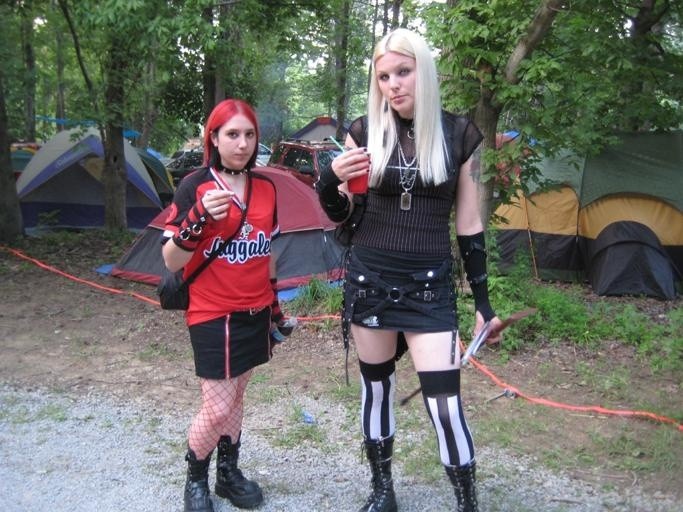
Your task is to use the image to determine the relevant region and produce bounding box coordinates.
[7,114,681,312]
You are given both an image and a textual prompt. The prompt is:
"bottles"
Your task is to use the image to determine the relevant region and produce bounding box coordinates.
[268,318,296,347]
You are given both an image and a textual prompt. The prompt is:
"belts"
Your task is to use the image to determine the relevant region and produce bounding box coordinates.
[233,304,269,317]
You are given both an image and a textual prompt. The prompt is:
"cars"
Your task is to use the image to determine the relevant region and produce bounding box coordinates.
[255,143,271,168]
[164,150,203,187]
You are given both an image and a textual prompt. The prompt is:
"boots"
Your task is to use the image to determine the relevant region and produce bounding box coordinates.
[183,438,216,512]
[442,456,480,512]
[215,430,262,509]
[355,433,398,512]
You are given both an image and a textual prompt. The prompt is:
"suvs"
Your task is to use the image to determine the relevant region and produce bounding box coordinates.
[265,137,344,191]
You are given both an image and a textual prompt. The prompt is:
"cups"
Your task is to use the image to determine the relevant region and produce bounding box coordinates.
[204,189,232,228]
[347,151,371,194]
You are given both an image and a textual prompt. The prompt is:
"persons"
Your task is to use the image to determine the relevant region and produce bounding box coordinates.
[316,26,504,511]
[159,98,293,511]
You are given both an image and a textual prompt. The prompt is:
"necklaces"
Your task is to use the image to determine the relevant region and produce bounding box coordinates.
[219,163,248,178]
[396,137,422,211]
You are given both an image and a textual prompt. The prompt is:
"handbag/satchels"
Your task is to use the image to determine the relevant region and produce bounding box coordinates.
[334,190,367,247]
[155,268,193,310]
